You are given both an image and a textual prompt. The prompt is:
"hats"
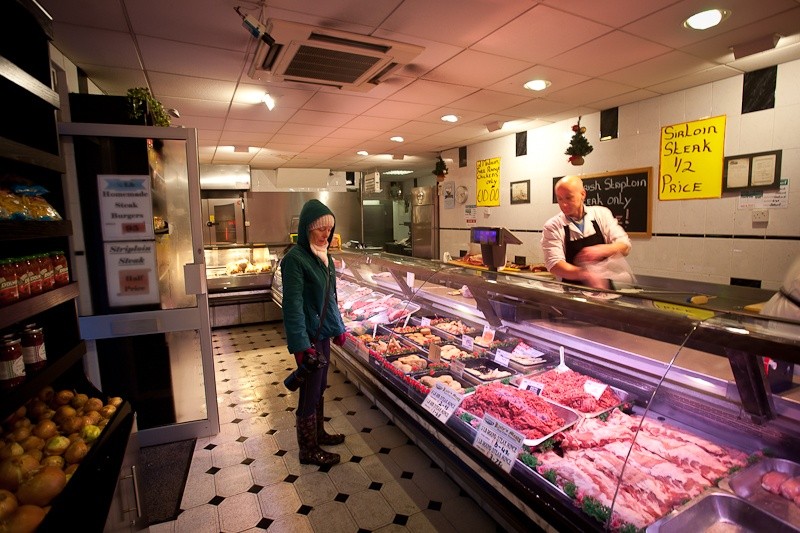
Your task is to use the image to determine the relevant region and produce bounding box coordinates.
[308,214,335,231]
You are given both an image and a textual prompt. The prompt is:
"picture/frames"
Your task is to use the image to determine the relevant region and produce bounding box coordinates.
[510,180,530,205]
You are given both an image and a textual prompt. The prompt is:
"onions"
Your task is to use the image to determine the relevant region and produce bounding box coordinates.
[0,388,122,533]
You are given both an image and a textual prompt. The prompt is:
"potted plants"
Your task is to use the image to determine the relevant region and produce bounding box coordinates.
[431,159,449,182]
[565,132,594,166]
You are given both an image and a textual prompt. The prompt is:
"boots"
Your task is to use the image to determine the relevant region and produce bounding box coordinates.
[316,397,345,446]
[296,408,341,466]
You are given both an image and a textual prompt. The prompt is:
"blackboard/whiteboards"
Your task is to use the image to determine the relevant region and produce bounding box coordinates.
[578,167,652,238]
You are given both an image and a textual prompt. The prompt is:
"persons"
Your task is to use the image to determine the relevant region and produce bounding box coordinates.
[760,259,800,335]
[282,199,346,466]
[541,176,633,293]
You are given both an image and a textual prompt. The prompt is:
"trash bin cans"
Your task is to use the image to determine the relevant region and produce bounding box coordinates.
[762,356,794,393]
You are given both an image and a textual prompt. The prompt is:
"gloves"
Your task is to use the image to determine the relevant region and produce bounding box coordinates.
[294,347,316,367]
[332,332,346,347]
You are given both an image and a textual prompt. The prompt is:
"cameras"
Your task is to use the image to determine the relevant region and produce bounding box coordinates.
[284,343,327,391]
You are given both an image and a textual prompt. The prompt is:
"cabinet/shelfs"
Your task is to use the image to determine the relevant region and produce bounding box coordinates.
[0,57,177,428]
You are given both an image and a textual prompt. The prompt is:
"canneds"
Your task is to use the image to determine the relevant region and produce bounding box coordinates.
[0,251,70,307]
[0,323,47,391]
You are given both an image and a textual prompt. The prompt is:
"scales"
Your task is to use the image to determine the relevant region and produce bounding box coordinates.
[470,227,564,323]
